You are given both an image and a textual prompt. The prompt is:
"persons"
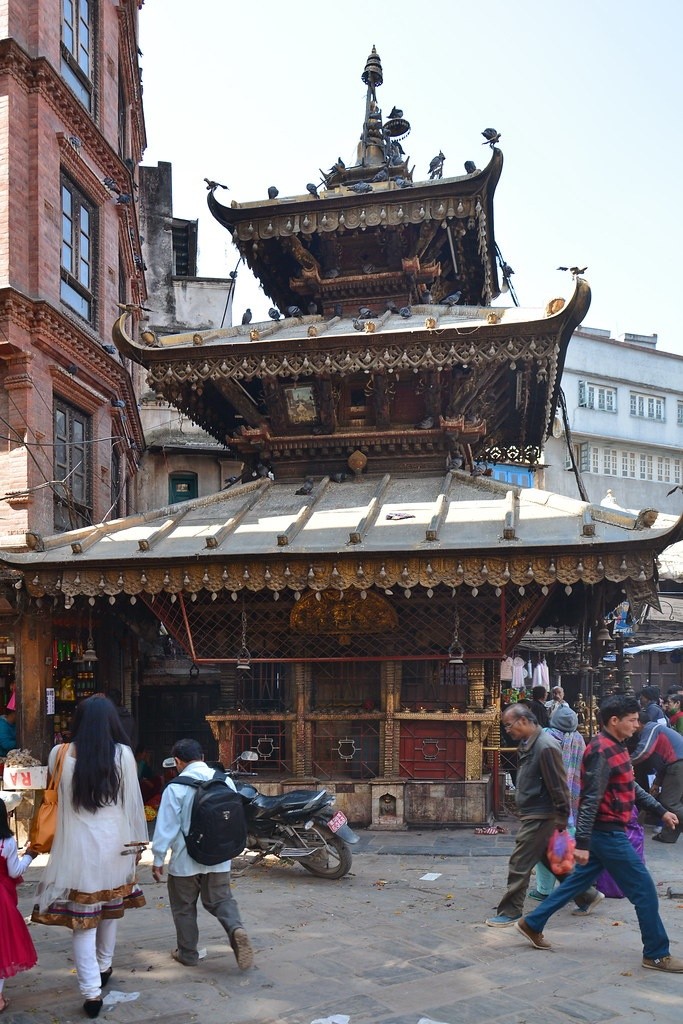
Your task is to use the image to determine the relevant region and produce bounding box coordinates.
[0,799,38,1010]
[499,684,683,916]
[30,696,149,1019]
[151,739,254,971]
[0,711,17,757]
[517,694,683,973]
[491,703,570,924]
[134,744,173,781]
[105,688,137,754]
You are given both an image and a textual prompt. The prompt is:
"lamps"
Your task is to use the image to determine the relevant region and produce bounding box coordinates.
[449,594,465,664]
[236,593,251,670]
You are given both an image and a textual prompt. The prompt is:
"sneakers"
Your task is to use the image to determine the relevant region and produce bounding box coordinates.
[642,955,683,973]
[486,914,522,928]
[572,891,605,916]
[514,916,551,949]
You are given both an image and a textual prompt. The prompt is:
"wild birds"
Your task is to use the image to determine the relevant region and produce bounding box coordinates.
[201,105,589,325]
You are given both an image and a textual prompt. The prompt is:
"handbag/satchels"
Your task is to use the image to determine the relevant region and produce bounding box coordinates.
[29,743,70,855]
[546,826,575,875]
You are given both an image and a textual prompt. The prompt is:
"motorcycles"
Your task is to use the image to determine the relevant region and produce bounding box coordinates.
[162,751,361,880]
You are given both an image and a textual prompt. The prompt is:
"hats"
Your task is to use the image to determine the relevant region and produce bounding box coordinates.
[550,704,578,732]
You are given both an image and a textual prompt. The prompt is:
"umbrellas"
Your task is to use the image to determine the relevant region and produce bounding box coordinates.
[623,639,683,686]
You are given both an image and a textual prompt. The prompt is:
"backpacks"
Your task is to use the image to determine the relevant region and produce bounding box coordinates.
[162,769,248,866]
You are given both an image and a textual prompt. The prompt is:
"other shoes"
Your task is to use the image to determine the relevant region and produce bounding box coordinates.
[230,927,253,970]
[652,832,664,843]
[0,994,11,1012]
[171,947,182,964]
[527,890,548,901]
[84,999,102,1018]
[100,966,112,988]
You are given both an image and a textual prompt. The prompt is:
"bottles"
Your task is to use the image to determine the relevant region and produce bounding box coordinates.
[0,632,96,746]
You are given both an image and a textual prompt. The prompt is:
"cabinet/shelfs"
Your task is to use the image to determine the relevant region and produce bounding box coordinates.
[56,659,99,711]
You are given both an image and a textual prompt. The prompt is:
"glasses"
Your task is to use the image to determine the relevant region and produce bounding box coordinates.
[503,715,523,729]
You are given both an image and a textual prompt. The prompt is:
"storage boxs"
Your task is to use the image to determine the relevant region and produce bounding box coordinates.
[3,766,49,790]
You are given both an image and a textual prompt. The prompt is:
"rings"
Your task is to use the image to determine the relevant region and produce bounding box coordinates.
[152,873,156,876]
